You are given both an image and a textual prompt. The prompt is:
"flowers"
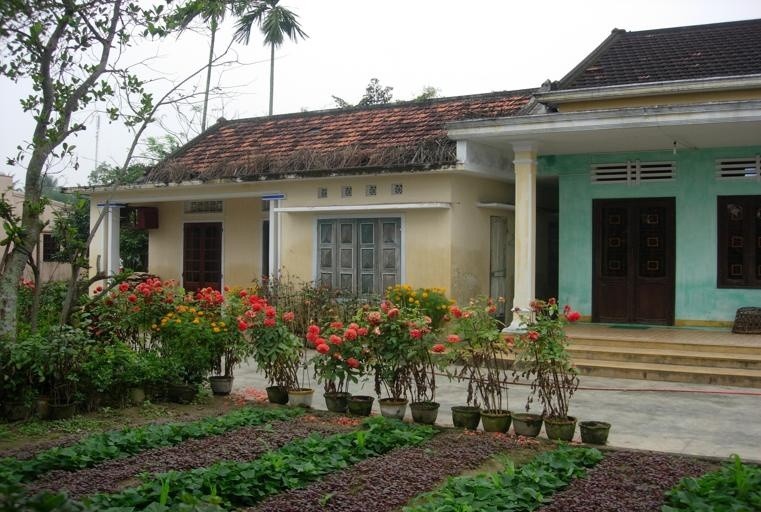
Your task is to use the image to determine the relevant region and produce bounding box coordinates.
[430,284,580,417]
[88,279,251,371]
[235,284,431,398]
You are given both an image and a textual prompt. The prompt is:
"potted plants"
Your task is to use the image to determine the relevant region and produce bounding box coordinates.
[0,319,91,424]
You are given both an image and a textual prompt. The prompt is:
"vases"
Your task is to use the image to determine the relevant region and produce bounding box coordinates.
[208,373,235,397]
[284,386,316,408]
[377,398,407,420]
[345,394,375,418]
[409,401,610,445]
[263,385,291,405]
[321,389,353,413]
